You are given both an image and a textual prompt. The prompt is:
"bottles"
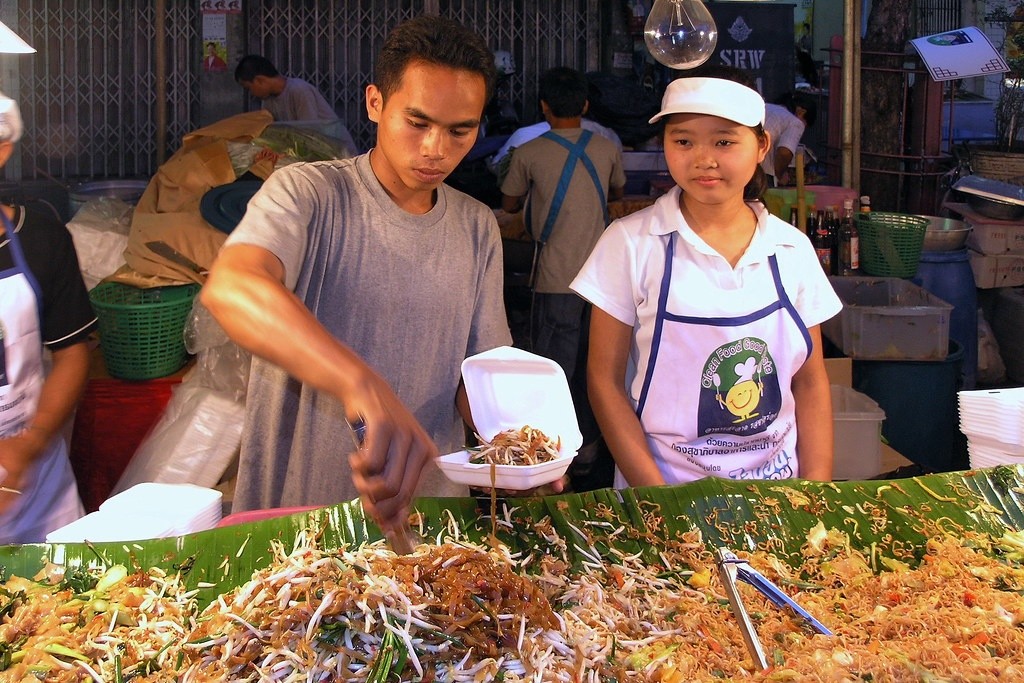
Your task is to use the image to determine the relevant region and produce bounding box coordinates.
[806,205,840,275]
[838,200,860,276]
[860,196,871,222]
[790,206,798,228]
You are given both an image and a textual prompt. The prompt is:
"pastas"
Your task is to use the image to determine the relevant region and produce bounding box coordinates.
[596,502,1024,683]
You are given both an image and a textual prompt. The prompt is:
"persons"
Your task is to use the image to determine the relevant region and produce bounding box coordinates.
[798,23,811,55]
[204,42,227,71]
[757,90,818,188]
[0,94,101,548]
[235,54,361,157]
[502,66,628,377]
[571,67,842,500]
[199,11,567,528]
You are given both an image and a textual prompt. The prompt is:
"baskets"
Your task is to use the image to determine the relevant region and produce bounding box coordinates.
[87,281,201,380]
[851,211,931,278]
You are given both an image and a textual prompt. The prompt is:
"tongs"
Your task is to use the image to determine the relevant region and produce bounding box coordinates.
[716,547,833,669]
[346,411,421,555]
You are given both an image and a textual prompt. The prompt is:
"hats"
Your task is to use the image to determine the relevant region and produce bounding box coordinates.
[650,77,766,127]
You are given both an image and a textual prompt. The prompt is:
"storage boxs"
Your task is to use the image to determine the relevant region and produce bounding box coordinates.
[622,135,668,171]
[965,215,1024,289]
[821,275,955,362]
[830,383,886,481]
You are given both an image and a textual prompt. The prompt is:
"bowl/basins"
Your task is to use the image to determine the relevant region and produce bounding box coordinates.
[970,194,1024,221]
[915,215,973,252]
[787,186,858,214]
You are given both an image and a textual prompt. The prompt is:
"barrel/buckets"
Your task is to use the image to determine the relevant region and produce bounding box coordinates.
[910,245,978,373]
[69,180,148,219]
[831,338,965,472]
